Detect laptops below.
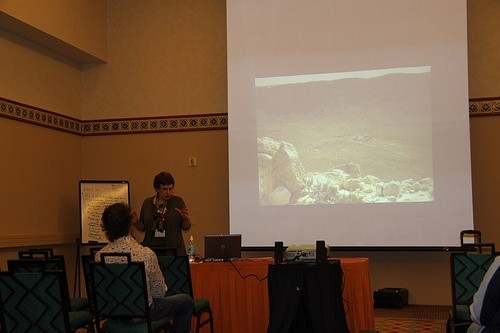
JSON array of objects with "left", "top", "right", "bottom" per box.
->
[{"left": 192, "top": 234, "right": 242, "bottom": 262}]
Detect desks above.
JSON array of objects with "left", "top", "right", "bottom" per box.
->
[{"left": 171, "top": 257, "right": 374, "bottom": 333}]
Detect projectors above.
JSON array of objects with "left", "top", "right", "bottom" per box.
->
[{"left": 285, "top": 243, "right": 329, "bottom": 260}]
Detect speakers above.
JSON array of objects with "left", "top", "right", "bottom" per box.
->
[{"left": 373, "top": 288, "right": 408, "bottom": 309}]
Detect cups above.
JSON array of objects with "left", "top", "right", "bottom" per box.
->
[
  {"left": 315, "top": 240, "right": 326, "bottom": 261},
  {"left": 274, "top": 241, "right": 283, "bottom": 263}
]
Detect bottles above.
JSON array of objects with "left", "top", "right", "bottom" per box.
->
[{"left": 188, "top": 235, "right": 195, "bottom": 262}]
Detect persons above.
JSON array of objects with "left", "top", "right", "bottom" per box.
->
[
  {"left": 131, "top": 171, "right": 191, "bottom": 255},
  {"left": 96, "top": 203, "right": 193, "bottom": 323},
  {"left": 466, "top": 256, "right": 500, "bottom": 333}
]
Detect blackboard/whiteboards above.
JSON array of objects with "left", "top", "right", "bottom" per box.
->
[{"left": 79, "top": 179, "right": 131, "bottom": 246}]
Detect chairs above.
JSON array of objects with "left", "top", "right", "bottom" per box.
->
[
  {"left": 0, "top": 249, "right": 211, "bottom": 333},
  {"left": 451, "top": 251, "right": 500, "bottom": 333}
]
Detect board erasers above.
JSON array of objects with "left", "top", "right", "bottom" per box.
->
[{"left": 89, "top": 240, "right": 98, "bottom": 244}]
